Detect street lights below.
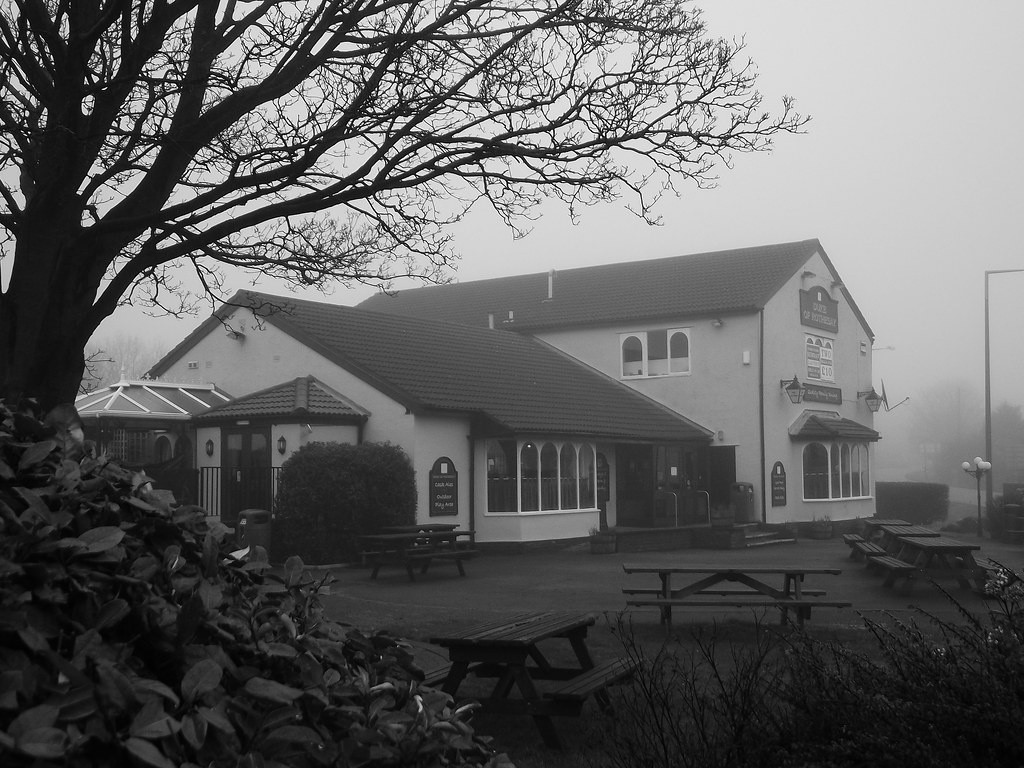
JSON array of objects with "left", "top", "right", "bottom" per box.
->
[{"left": 961, "top": 456, "right": 992, "bottom": 537}]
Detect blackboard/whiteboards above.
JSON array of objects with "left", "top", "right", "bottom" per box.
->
[
  {"left": 771, "top": 461, "right": 787, "bottom": 507},
  {"left": 429, "top": 456, "right": 458, "bottom": 518},
  {"left": 588, "top": 452, "right": 610, "bottom": 501}
]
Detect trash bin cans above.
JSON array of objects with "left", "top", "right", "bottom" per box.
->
[
  {"left": 730, "top": 481, "right": 755, "bottom": 523},
  {"left": 237, "top": 508, "right": 272, "bottom": 563}
]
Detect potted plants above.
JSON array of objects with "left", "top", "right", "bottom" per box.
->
[
  {"left": 810, "top": 515, "right": 833, "bottom": 540},
  {"left": 588, "top": 525, "right": 617, "bottom": 553}
]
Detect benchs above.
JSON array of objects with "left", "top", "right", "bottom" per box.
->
[
  {"left": 546, "top": 654, "right": 647, "bottom": 704},
  {"left": 842, "top": 534, "right": 865, "bottom": 543},
  {"left": 955, "top": 556, "right": 998, "bottom": 572},
  {"left": 622, "top": 586, "right": 826, "bottom": 595},
  {"left": 359, "top": 539, "right": 479, "bottom": 566},
  {"left": 626, "top": 598, "right": 851, "bottom": 609},
  {"left": 854, "top": 542, "right": 887, "bottom": 556},
  {"left": 869, "top": 555, "right": 918, "bottom": 573},
  {"left": 422, "top": 660, "right": 486, "bottom": 687}
]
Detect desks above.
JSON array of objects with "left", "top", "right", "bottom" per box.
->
[
  {"left": 865, "top": 525, "right": 941, "bottom": 579},
  {"left": 364, "top": 530, "right": 477, "bottom": 584},
  {"left": 378, "top": 523, "right": 461, "bottom": 562},
  {"left": 850, "top": 518, "right": 913, "bottom": 562},
  {"left": 622, "top": 562, "right": 842, "bottom": 630},
  {"left": 882, "top": 536, "right": 986, "bottom": 596},
  {"left": 430, "top": 610, "right": 615, "bottom": 751}
]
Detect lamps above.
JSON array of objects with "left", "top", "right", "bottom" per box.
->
[
  {"left": 857, "top": 387, "right": 883, "bottom": 412},
  {"left": 780, "top": 375, "right": 806, "bottom": 404},
  {"left": 226, "top": 330, "right": 245, "bottom": 339},
  {"left": 277, "top": 436, "right": 286, "bottom": 455},
  {"left": 711, "top": 315, "right": 723, "bottom": 327},
  {"left": 206, "top": 438, "right": 214, "bottom": 457}
]
